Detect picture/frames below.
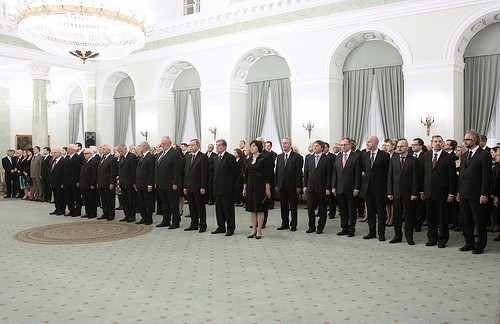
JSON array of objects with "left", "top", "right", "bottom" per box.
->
[{"left": 85, "top": 132, "right": 97, "bottom": 148}]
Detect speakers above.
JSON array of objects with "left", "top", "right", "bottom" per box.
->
[{"left": 85, "top": 132, "right": 96, "bottom": 148}]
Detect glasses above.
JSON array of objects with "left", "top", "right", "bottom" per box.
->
[
  {"left": 464, "top": 138, "right": 472, "bottom": 141},
  {"left": 84, "top": 153, "right": 89, "bottom": 155}
]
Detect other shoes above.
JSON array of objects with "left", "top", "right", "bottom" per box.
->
[
  {"left": 47, "top": 201, "right": 364, "bottom": 239},
  {"left": 362, "top": 225, "right": 500, "bottom": 254},
  {"left": 3, "top": 195, "right": 55, "bottom": 203}
]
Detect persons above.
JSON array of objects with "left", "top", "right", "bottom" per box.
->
[{"left": 2, "top": 129, "right": 500, "bottom": 254}]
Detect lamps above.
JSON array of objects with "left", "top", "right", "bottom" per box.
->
[
  {"left": 302, "top": 123, "right": 315, "bottom": 138},
  {"left": 141, "top": 131, "right": 147, "bottom": 142},
  {"left": 421, "top": 115, "right": 434, "bottom": 135},
  {"left": 0, "top": 0, "right": 157, "bottom": 65},
  {"left": 209, "top": 127, "right": 217, "bottom": 142}
]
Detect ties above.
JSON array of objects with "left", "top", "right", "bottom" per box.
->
[
  {"left": 343, "top": 153, "right": 347, "bottom": 168},
  {"left": 415, "top": 154, "right": 417, "bottom": 158},
  {"left": 86, "top": 160, "right": 88, "bottom": 163},
  {"left": 432, "top": 153, "right": 438, "bottom": 170},
  {"left": 140, "top": 155, "right": 143, "bottom": 162},
  {"left": 191, "top": 155, "right": 195, "bottom": 165},
  {"left": 102, "top": 156, "right": 106, "bottom": 161},
  {"left": 219, "top": 155, "right": 222, "bottom": 164},
  {"left": 54, "top": 159, "right": 57, "bottom": 165},
  {"left": 400, "top": 156, "right": 406, "bottom": 171},
  {"left": 315, "top": 155, "right": 319, "bottom": 168},
  {"left": 159, "top": 152, "right": 165, "bottom": 161},
  {"left": 284, "top": 154, "right": 288, "bottom": 166},
  {"left": 370, "top": 152, "right": 374, "bottom": 166},
  {"left": 468, "top": 151, "right": 472, "bottom": 159}
]
